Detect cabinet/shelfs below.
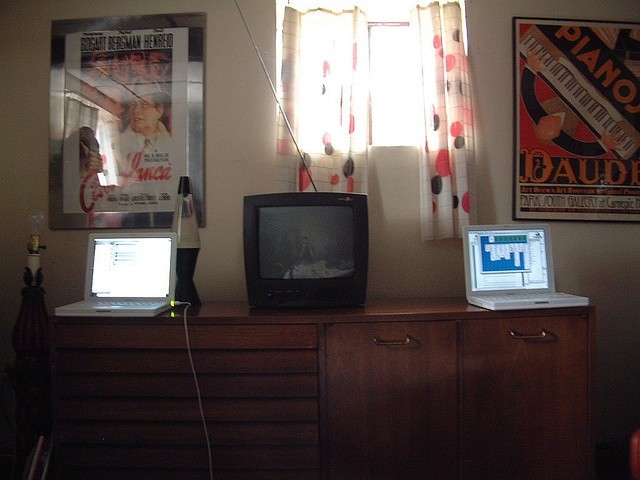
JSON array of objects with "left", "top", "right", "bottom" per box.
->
[
  {"left": 320, "top": 307, "right": 595, "bottom": 478},
  {"left": 52, "top": 318, "right": 319, "bottom": 474}
]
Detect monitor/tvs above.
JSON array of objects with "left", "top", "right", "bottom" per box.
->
[{"left": 244, "top": 193, "right": 370, "bottom": 304}]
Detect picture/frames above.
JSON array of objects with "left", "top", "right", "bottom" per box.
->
[
  {"left": 48, "top": 13, "right": 207, "bottom": 230},
  {"left": 512, "top": 17, "right": 639, "bottom": 224}
]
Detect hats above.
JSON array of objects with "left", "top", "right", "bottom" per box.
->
[{"left": 120, "top": 78, "right": 171, "bottom": 108}]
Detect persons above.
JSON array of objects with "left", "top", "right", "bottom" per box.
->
[
  {"left": 113, "top": 78, "right": 172, "bottom": 157},
  {"left": 93, "top": 55, "right": 113, "bottom": 78},
  {"left": 298, "top": 237, "right": 316, "bottom": 264},
  {"left": 127, "top": 50, "right": 144, "bottom": 73},
  {"left": 149, "top": 54, "right": 170, "bottom": 77}
]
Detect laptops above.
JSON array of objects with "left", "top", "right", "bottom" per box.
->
[
  {"left": 455, "top": 208, "right": 590, "bottom": 310},
  {"left": 54, "top": 235, "right": 182, "bottom": 322}
]
